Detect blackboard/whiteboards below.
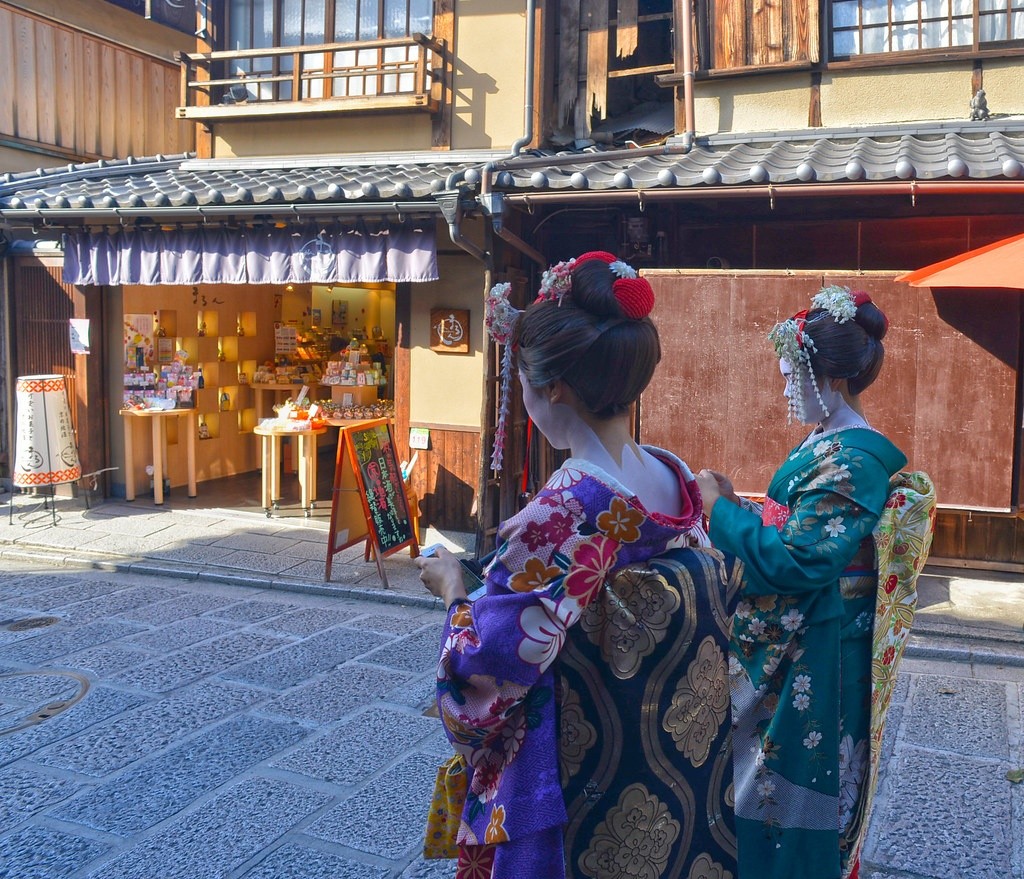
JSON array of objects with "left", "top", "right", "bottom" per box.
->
[{"left": 324, "top": 417, "right": 421, "bottom": 558}]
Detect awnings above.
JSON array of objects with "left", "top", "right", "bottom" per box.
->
[{"left": 894, "top": 234, "right": 1024, "bottom": 289}]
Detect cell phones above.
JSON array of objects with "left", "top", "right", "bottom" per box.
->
[{"left": 422, "top": 543, "right": 487, "bottom": 603}]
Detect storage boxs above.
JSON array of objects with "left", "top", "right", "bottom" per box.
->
[{"left": 331, "top": 385, "right": 377, "bottom": 409}]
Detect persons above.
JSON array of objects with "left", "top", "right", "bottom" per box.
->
[
  {"left": 414, "top": 251, "right": 750, "bottom": 879},
  {"left": 696, "top": 285, "right": 936, "bottom": 879}
]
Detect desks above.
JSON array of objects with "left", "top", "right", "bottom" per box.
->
[
  {"left": 249, "top": 382, "right": 396, "bottom": 518},
  {"left": 119, "top": 407, "right": 199, "bottom": 506}
]
